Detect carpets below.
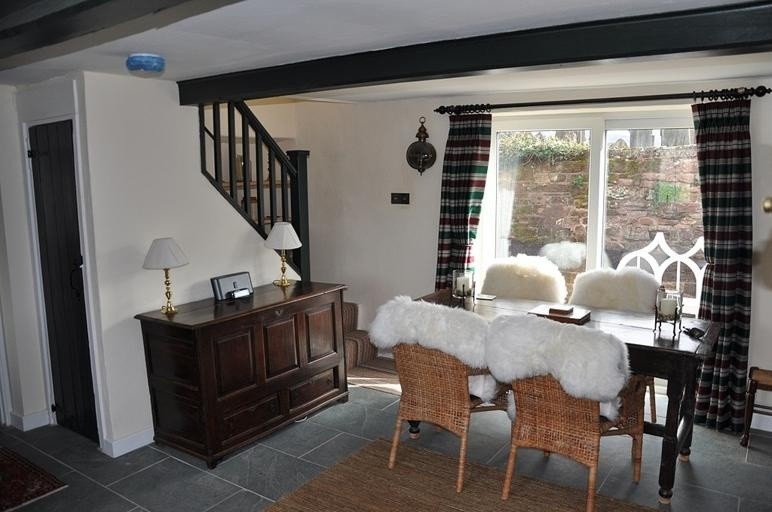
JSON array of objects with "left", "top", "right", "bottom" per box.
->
[
  {"left": 0, "top": 443, "right": 68, "bottom": 510},
  {"left": 263, "top": 437, "right": 633, "bottom": 512}
]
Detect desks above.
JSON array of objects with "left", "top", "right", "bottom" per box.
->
[{"left": 407, "top": 289, "right": 722, "bottom": 505}]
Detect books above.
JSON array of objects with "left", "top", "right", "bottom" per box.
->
[{"left": 527, "top": 304, "right": 591, "bottom": 325}]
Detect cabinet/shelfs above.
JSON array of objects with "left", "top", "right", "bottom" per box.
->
[{"left": 135, "top": 280, "right": 351, "bottom": 469}]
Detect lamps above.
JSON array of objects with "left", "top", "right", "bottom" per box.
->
[
  {"left": 143, "top": 238, "right": 189, "bottom": 315},
  {"left": 264, "top": 220, "right": 303, "bottom": 285},
  {"left": 406, "top": 118, "right": 435, "bottom": 174}
]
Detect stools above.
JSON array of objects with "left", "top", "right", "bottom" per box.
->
[{"left": 740, "top": 367, "right": 772, "bottom": 447}]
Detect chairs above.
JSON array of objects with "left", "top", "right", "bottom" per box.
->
[
  {"left": 487, "top": 312, "right": 647, "bottom": 511},
  {"left": 482, "top": 259, "right": 568, "bottom": 303},
  {"left": 369, "top": 296, "right": 516, "bottom": 494},
  {"left": 571, "top": 267, "right": 663, "bottom": 423}
]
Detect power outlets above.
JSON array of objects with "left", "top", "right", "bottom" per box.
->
[{"left": 391, "top": 192, "right": 409, "bottom": 204}]
